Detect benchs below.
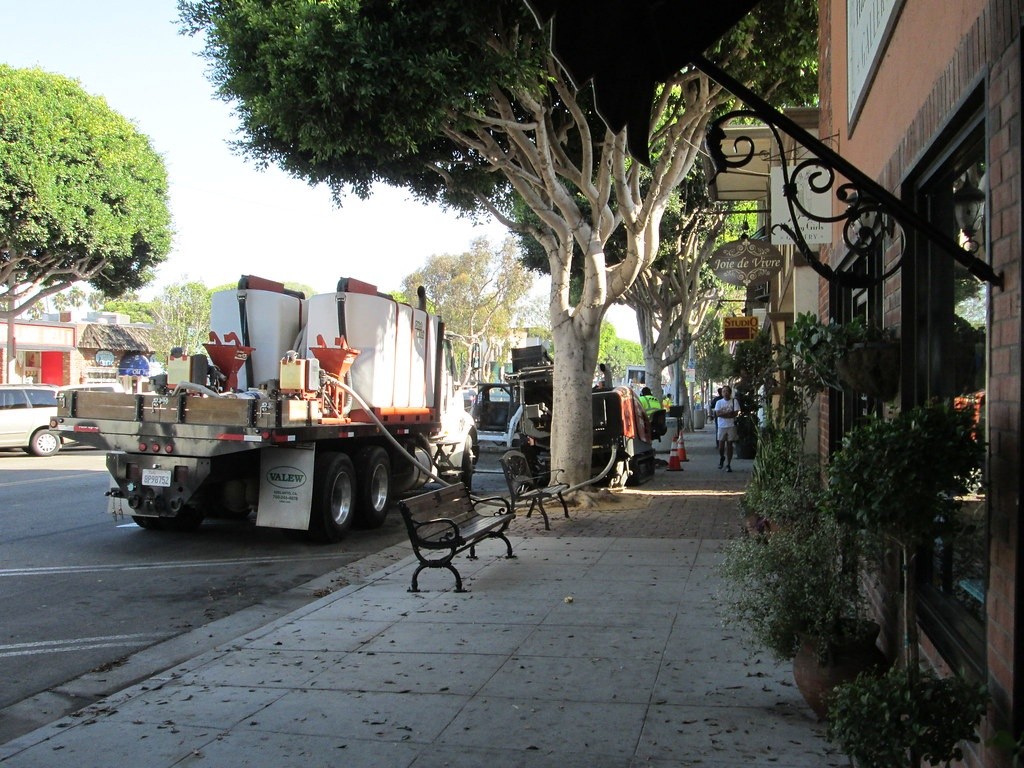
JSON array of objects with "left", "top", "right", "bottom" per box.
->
[
  {"left": 499, "top": 449, "right": 570, "bottom": 531},
  {"left": 397, "top": 482, "right": 516, "bottom": 592}
]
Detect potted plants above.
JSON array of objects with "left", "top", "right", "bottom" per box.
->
[
  {"left": 744, "top": 484, "right": 819, "bottom": 545},
  {"left": 772, "top": 310, "right": 901, "bottom": 399},
  {"left": 712, "top": 538, "right": 887, "bottom": 720}
]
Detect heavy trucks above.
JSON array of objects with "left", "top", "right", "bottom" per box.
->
[{"left": 47, "top": 275, "right": 481, "bottom": 545}]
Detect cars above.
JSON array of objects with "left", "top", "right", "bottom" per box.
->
[
  {"left": 0, "top": 384, "right": 83, "bottom": 459},
  {"left": 458, "top": 340, "right": 679, "bottom": 496},
  {"left": 56, "top": 380, "right": 129, "bottom": 407}
]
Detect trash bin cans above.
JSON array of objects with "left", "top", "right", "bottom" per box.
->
[
  {"left": 694, "top": 409, "right": 704, "bottom": 429},
  {"left": 651, "top": 416, "right": 678, "bottom": 454}
]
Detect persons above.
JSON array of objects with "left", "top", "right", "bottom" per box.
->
[
  {"left": 694, "top": 393, "right": 701, "bottom": 409},
  {"left": 661, "top": 389, "right": 672, "bottom": 416},
  {"left": 714, "top": 386, "right": 742, "bottom": 472},
  {"left": 639, "top": 387, "right": 661, "bottom": 422},
  {"left": 711, "top": 388, "right": 723, "bottom": 449}
]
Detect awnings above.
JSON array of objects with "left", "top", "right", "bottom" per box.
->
[{"left": 520, "top": 0, "right": 1006, "bottom": 293}]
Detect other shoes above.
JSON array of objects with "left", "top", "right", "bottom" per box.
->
[
  {"left": 725, "top": 466, "right": 731, "bottom": 472},
  {"left": 718, "top": 455, "right": 725, "bottom": 469}
]
